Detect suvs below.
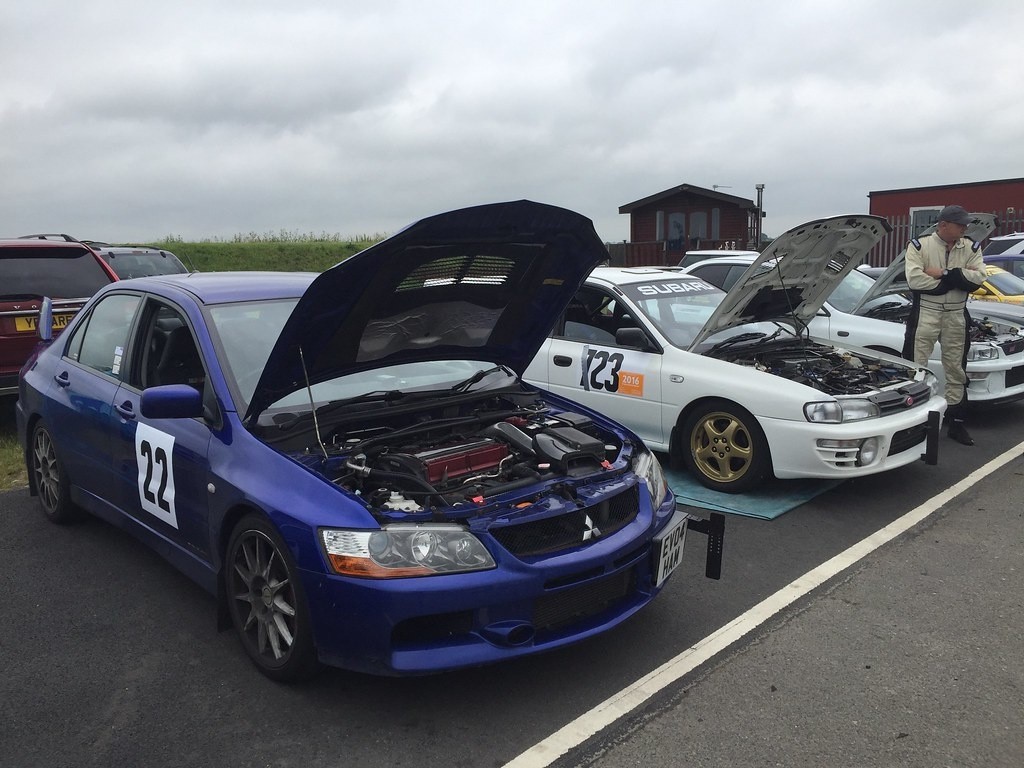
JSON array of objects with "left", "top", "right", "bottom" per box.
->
[{"left": 0, "top": 232, "right": 129, "bottom": 398}]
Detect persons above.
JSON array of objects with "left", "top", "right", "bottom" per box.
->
[{"left": 902, "top": 205, "right": 989, "bottom": 446}]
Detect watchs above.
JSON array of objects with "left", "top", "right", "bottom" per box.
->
[{"left": 942, "top": 269, "right": 949, "bottom": 276}]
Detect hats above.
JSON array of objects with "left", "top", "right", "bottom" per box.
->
[{"left": 936, "top": 205, "right": 974, "bottom": 225}]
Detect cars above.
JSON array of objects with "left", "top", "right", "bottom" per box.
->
[
  {"left": 13, "top": 198, "right": 691, "bottom": 687},
  {"left": 861, "top": 232, "right": 1024, "bottom": 308},
  {"left": 83, "top": 239, "right": 189, "bottom": 282},
  {"left": 423, "top": 212, "right": 949, "bottom": 495},
  {"left": 663, "top": 211, "right": 1024, "bottom": 418},
  {"left": 674, "top": 248, "right": 757, "bottom": 276}
]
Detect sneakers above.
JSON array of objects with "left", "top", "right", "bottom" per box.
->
[{"left": 947, "top": 424, "right": 974, "bottom": 445}]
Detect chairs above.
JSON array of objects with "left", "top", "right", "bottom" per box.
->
[{"left": 155, "top": 326, "right": 205, "bottom": 392}]
